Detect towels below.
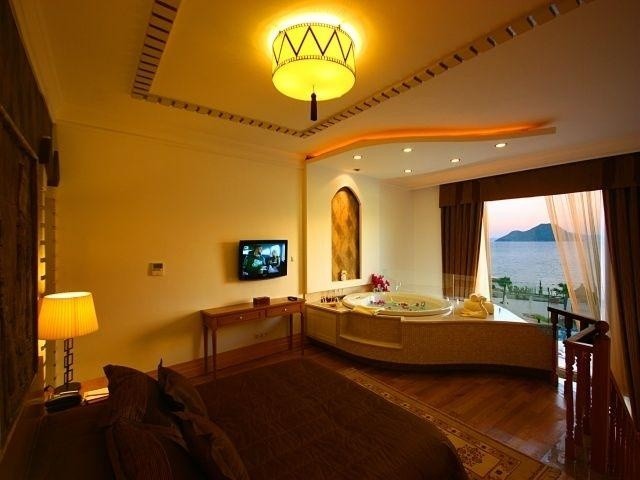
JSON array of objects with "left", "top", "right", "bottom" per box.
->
[{"left": 454, "top": 293, "right": 494, "bottom": 319}]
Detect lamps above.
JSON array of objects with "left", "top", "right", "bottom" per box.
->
[
  {"left": 268, "top": 22, "right": 362, "bottom": 122},
  {"left": 38, "top": 290, "right": 99, "bottom": 395}
]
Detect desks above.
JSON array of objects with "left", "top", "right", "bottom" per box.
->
[{"left": 199, "top": 295, "right": 307, "bottom": 382}]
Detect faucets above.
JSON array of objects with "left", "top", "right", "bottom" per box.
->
[
  {"left": 444, "top": 295, "right": 449, "bottom": 302},
  {"left": 454, "top": 297, "right": 460, "bottom": 305}
]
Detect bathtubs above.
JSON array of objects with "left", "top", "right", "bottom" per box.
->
[{"left": 342, "top": 291, "right": 451, "bottom": 316}]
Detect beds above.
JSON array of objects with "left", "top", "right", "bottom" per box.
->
[{"left": 1, "top": 355, "right": 468, "bottom": 480}]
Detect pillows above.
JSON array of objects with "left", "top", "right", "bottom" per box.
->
[{"left": 96, "top": 357, "right": 249, "bottom": 480}]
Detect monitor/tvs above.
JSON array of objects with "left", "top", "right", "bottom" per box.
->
[{"left": 238, "top": 239, "right": 288, "bottom": 280}]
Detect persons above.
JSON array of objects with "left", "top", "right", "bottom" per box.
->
[
  {"left": 243, "top": 245, "right": 268, "bottom": 275},
  {"left": 269, "top": 250, "right": 282, "bottom": 269}
]
